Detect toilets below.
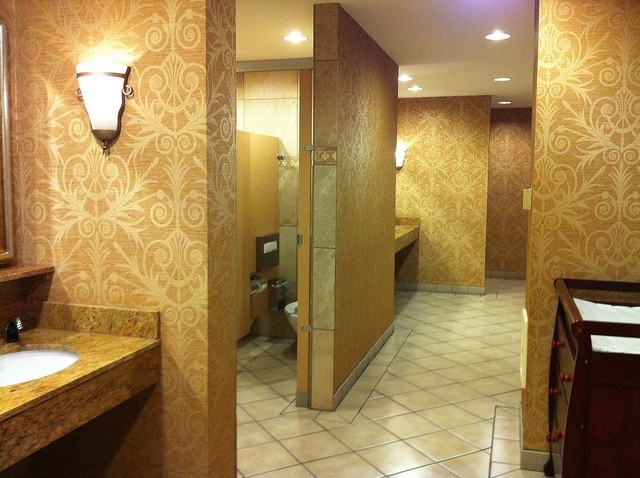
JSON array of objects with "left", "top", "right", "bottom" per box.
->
[{"left": 284, "top": 300, "right": 297, "bottom": 337}]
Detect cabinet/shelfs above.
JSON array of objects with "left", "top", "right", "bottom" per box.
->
[{"left": 543, "top": 278, "right": 640, "bottom": 477}]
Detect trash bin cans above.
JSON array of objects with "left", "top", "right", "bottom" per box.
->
[{"left": 268, "top": 278, "right": 289, "bottom": 312}]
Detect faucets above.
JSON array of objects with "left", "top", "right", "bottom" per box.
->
[{"left": 5, "top": 315, "right": 24, "bottom": 343}]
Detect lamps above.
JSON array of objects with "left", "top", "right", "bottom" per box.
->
[
  {"left": 395, "top": 150, "right": 406, "bottom": 170},
  {"left": 75, "top": 59, "right": 131, "bottom": 156}
]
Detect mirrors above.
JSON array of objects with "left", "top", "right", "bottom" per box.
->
[{"left": 0, "top": 25, "right": 15, "bottom": 266}]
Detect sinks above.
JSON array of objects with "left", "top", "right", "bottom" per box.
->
[{"left": 0, "top": 343, "right": 80, "bottom": 389}]
